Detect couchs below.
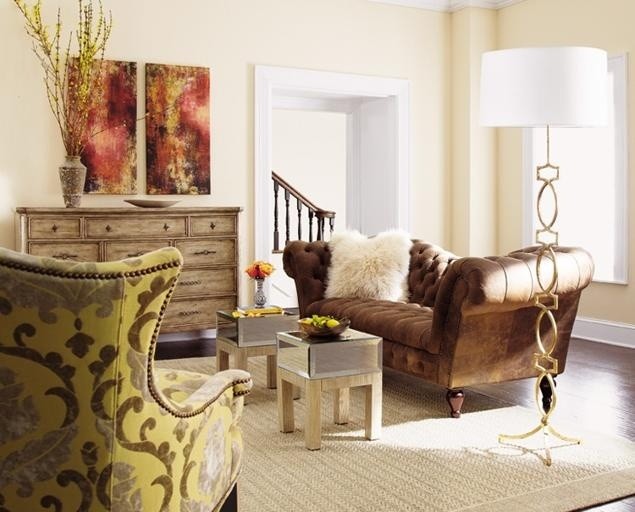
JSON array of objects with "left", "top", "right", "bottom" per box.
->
[{"left": 283, "top": 235, "right": 594, "bottom": 418}]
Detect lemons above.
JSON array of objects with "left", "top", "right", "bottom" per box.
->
[
  {"left": 326, "top": 320, "right": 340, "bottom": 328},
  {"left": 301, "top": 319, "right": 312, "bottom": 328}
]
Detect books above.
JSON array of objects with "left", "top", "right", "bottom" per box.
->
[{"left": 238, "top": 304, "right": 284, "bottom": 315}]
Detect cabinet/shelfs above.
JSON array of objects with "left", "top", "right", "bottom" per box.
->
[{"left": 16, "top": 206, "right": 244, "bottom": 335}]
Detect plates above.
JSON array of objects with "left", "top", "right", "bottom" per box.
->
[{"left": 123, "top": 200, "right": 180, "bottom": 208}]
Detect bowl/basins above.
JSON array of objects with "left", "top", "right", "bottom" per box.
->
[{"left": 297, "top": 317, "right": 351, "bottom": 338}]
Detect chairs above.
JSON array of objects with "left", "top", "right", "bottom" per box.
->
[{"left": 0, "top": 246, "right": 253, "bottom": 512}]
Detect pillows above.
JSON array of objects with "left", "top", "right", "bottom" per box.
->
[{"left": 324, "top": 228, "right": 411, "bottom": 305}]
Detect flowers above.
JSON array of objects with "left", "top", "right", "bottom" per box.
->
[
  {"left": 244, "top": 261, "right": 274, "bottom": 280},
  {"left": 14, "top": 0, "right": 184, "bottom": 156}
]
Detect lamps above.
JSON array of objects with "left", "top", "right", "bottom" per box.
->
[{"left": 480, "top": 46, "right": 608, "bottom": 464}]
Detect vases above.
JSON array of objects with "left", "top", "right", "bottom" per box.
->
[
  {"left": 255, "top": 280, "right": 266, "bottom": 308},
  {"left": 58, "top": 156, "right": 88, "bottom": 208}
]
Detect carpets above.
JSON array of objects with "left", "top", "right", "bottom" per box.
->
[{"left": 152, "top": 341, "right": 635, "bottom": 512}]
